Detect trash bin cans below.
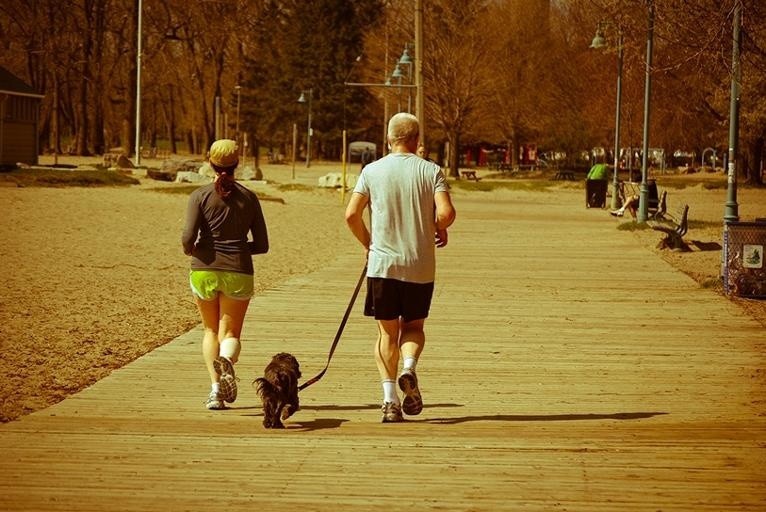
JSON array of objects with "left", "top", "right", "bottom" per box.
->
[{"left": 725, "top": 221, "right": 766, "bottom": 300}]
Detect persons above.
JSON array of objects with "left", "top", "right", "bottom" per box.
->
[
  {"left": 417, "top": 142, "right": 452, "bottom": 190},
  {"left": 361, "top": 147, "right": 372, "bottom": 168},
  {"left": 345, "top": 112, "right": 456, "bottom": 422},
  {"left": 585, "top": 162, "right": 610, "bottom": 208},
  {"left": 609, "top": 176, "right": 659, "bottom": 222},
  {"left": 181, "top": 139, "right": 268, "bottom": 408}
]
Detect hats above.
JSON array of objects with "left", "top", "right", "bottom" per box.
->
[{"left": 209, "top": 139, "right": 239, "bottom": 168}]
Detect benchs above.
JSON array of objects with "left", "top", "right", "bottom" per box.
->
[
  {"left": 555, "top": 171, "right": 575, "bottom": 181},
  {"left": 461, "top": 171, "right": 478, "bottom": 179},
  {"left": 606, "top": 182, "right": 693, "bottom": 252}
]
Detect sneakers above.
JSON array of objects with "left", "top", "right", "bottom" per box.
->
[
  {"left": 213, "top": 356, "right": 237, "bottom": 403},
  {"left": 206, "top": 393, "right": 225, "bottom": 409},
  {"left": 381, "top": 403, "right": 403, "bottom": 422},
  {"left": 398, "top": 371, "right": 422, "bottom": 415}
]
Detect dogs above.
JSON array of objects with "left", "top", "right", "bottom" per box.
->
[{"left": 251, "top": 351, "right": 302, "bottom": 430}]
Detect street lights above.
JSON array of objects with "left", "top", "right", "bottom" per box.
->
[
  {"left": 590, "top": 19, "right": 626, "bottom": 216},
  {"left": 392, "top": 63, "right": 410, "bottom": 113},
  {"left": 399, "top": 44, "right": 413, "bottom": 116},
  {"left": 384, "top": 77, "right": 396, "bottom": 86},
  {"left": 297, "top": 88, "right": 314, "bottom": 170}
]
